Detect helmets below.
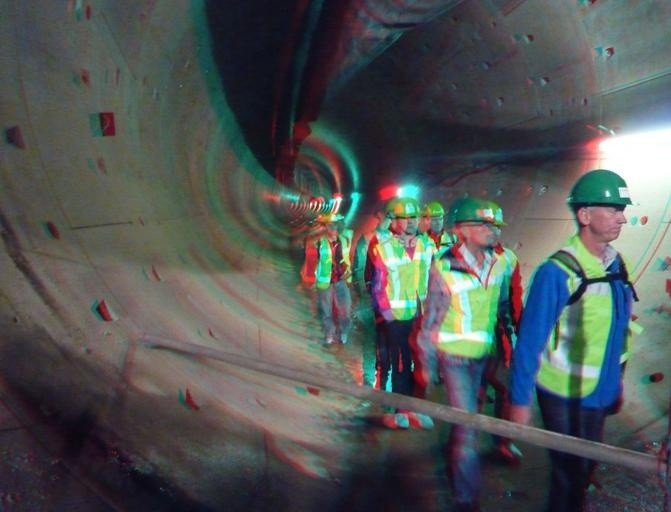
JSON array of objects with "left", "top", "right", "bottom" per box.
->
[
  {"left": 566, "top": 170, "right": 632, "bottom": 205},
  {"left": 319, "top": 213, "right": 344, "bottom": 223},
  {"left": 373, "top": 197, "right": 506, "bottom": 225}
]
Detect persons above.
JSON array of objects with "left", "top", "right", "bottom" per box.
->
[{"left": 299, "top": 169, "right": 639, "bottom": 511}]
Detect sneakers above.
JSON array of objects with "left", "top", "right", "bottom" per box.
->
[
  {"left": 498, "top": 440, "right": 523, "bottom": 461},
  {"left": 326, "top": 334, "right": 348, "bottom": 344},
  {"left": 384, "top": 412, "right": 434, "bottom": 428}
]
